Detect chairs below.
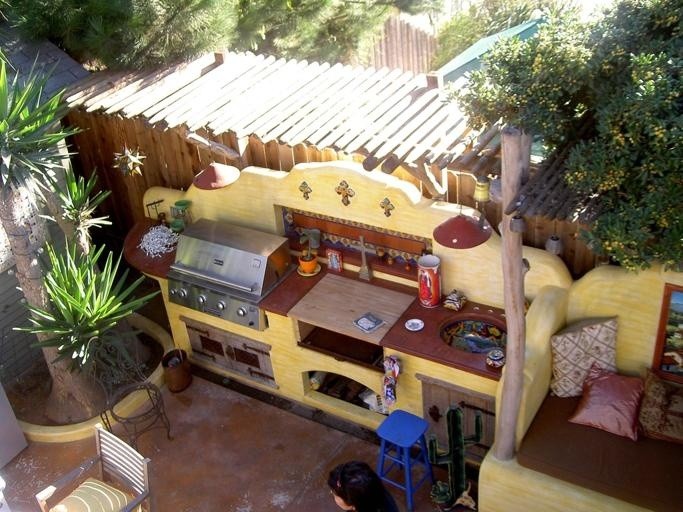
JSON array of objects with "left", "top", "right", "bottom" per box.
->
[{"left": 34, "top": 422, "right": 156, "bottom": 512}]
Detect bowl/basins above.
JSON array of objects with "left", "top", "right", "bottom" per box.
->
[{"left": 169, "top": 220, "right": 183, "bottom": 232}]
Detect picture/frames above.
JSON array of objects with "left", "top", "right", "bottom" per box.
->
[{"left": 650, "top": 282, "right": 683, "bottom": 387}]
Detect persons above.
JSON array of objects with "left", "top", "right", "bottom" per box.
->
[{"left": 328, "top": 460, "right": 399, "bottom": 511}]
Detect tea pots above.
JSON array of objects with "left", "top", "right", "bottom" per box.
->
[
  {"left": 486, "top": 350, "right": 505, "bottom": 370},
  {"left": 417, "top": 255, "right": 441, "bottom": 310}
]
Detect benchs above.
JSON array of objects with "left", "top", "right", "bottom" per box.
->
[{"left": 477, "top": 254, "right": 682, "bottom": 511}]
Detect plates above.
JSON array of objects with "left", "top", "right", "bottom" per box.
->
[{"left": 404, "top": 317, "right": 424, "bottom": 333}]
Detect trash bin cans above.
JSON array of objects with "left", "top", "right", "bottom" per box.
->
[{"left": 161, "top": 349, "right": 192, "bottom": 394}]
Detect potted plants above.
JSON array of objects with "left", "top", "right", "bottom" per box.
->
[{"left": 298, "top": 226, "right": 322, "bottom": 276}]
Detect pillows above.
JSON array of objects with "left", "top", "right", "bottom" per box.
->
[{"left": 552, "top": 313, "right": 683, "bottom": 444}]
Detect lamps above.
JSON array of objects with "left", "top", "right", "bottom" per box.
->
[
  {"left": 432, "top": 175, "right": 491, "bottom": 248},
  {"left": 193, "top": 127, "right": 241, "bottom": 190}
]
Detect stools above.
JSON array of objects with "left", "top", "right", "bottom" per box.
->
[{"left": 375, "top": 410, "right": 437, "bottom": 512}]
